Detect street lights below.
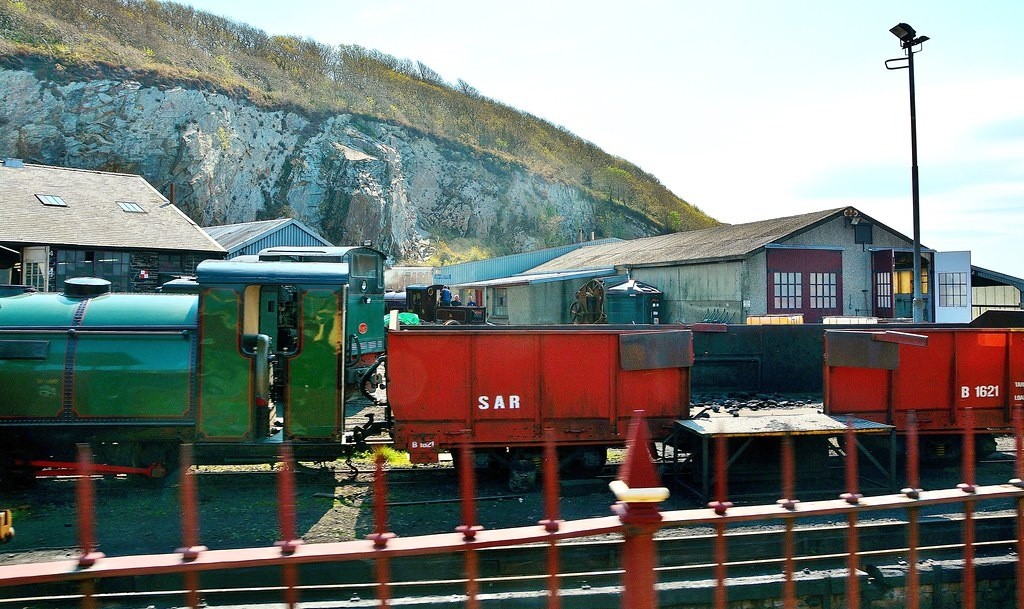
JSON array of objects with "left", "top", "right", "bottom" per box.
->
[{"left": 883, "top": 22, "right": 930, "bottom": 326}]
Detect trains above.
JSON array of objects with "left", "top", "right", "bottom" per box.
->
[
  {"left": 384, "top": 284, "right": 488, "bottom": 325},
  {"left": 1, "top": 257, "right": 1023, "bottom": 490}
]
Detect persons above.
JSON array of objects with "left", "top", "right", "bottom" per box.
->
[
  {"left": 467, "top": 295, "right": 476, "bottom": 307},
  {"left": 451, "top": 294, "right": 462, "bottom": 306},
  {"left": 438, "top": 285, "right": 452, "bottom": 307}
]
